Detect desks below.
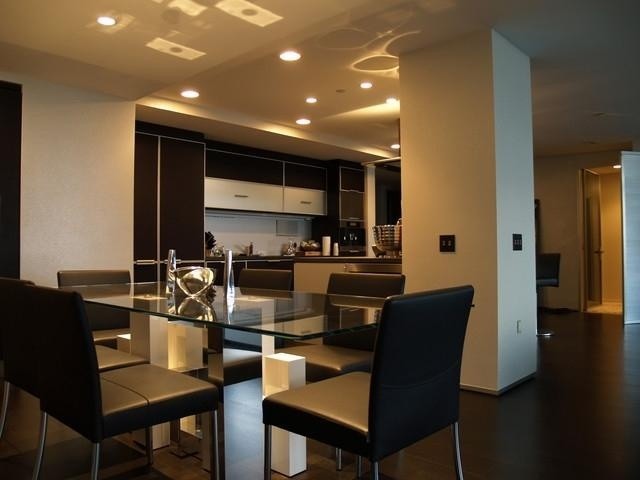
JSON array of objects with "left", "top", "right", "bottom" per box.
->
[{"left": 79, "top": 286, "right": 408, "bottom": 479}]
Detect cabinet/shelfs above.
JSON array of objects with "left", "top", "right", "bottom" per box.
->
[
  {"left": 206, "top": 146, "right": 284, "bottom": 214},
  {"left": 310, "top": 159, "right": 365, "bottom": 253},
  {"left": 134, "top": 132, "right": 206, "bottom": 283},
  {"left": 283, "top": 157, "right": 328, "bottom": 218}
]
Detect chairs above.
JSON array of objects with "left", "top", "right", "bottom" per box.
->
[
  {"left": 537, "top": 249, "right": 562, "bottom": 338},
  {"left": 54, "top": 265, "right": 138, "bottom": 345},
  {"left": 274, "top": 270, "right": 408, "bottom": 471},
  {"left": 29, "top": 287, "right": 223, "bottom": 479},
  {"left": 261, "top": 285, "right": 480, "bottom": 479},
  {"left": 1, "top": 277, "right": 147, "bottom": 478},
  {"left": 207, "top": 267, "right": 293, "bottom": 387}
]
